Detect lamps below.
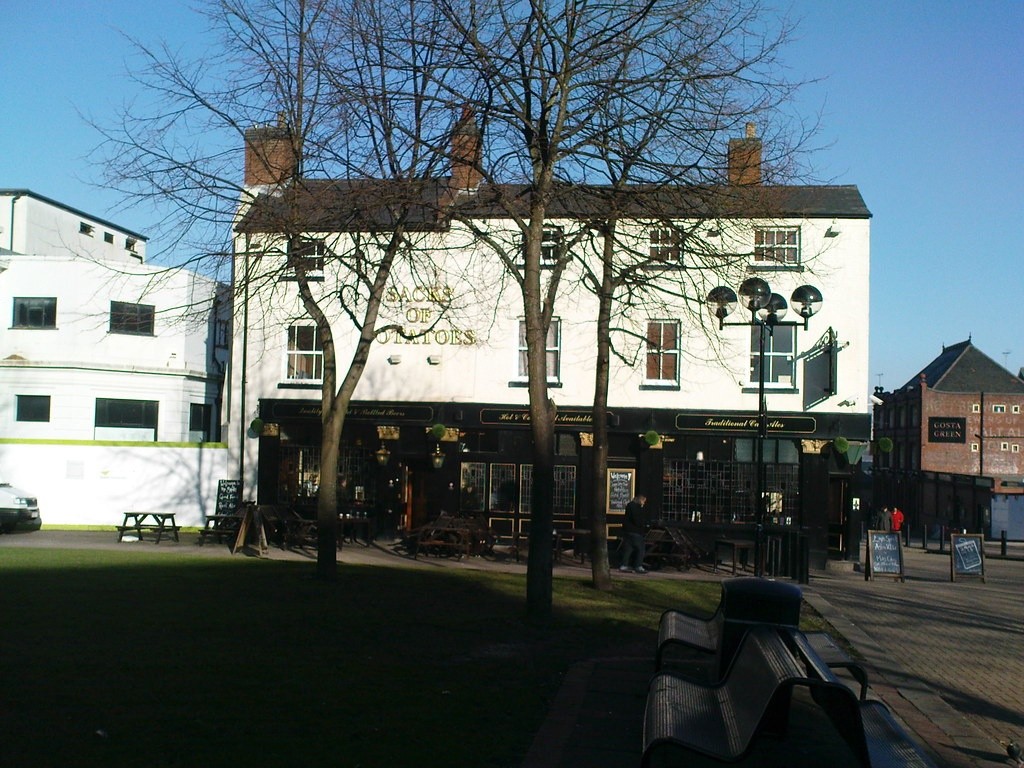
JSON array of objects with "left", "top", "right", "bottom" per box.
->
[
  {"left": 391, "top": 355, "right": 401, "bottom": 363},
  {"left": 429, "top": 355, "right": 442, "bottom": 364},
  {"left": 432, "top": 442, "right": 446, "bottom": 469},
  {"left": 376, "top": 440, "right": 391, "bottom": 466}
]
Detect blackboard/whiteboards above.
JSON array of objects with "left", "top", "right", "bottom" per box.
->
[
  {"left": 949, "top": 533, "right": 985, "bottom": 578},
  {"left": 607, "top": 466, "right": 636, "bottom": 515},
  {"left": 866, "top": 530, "right": 906, "bottom": 577},
  {"left": 215, "top": 478, "right": 242, "bottom": 518}
]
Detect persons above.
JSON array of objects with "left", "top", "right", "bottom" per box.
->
[
  {"left": 618, "top": 494, "right": 650, "bottom": 574},
  {"left": 875, "top": 505, "right": 893, "bottom": 531},
  {"left": 889, "top": 506, "right": 904, "bottom": 531}
]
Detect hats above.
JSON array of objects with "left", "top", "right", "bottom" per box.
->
[{"left": 635, "top": 492, "right": 648, "bottom": 498}]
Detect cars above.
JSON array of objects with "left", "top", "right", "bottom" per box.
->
[{"left": 1, "top": 481, "right": 41, "bottom": 534}]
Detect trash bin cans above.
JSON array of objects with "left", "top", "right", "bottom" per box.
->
[
  {"left": 764, "top": 528, "right": 812, "bottom": 578},
  {"left": 715, "top": 579, "right": 801, "bottom": 698}
]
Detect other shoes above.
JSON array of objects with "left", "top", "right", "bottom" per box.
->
[{"left": 617, "top": 564, "right": 648, "bottom": 573}]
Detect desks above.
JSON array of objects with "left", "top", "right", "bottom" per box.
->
[
  {"left": 512, "top": 532, "right": 529, "bottom": 562},
  {"left": 714, "top": 540, "right": 756, "bottom": 575},
  {"left": 199, "top": 515, "right": 243, "bottom": 546},
  {"left": 556, "top": 529, "right": 591, "bottom": 564},
  {"left": 118, "top": 513, "right": 180, "bottom": 544},
  {"left": 337, "top": 518, "right": 373, "bottom": 550}
]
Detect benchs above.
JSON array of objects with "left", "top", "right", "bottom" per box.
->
[
  {"left": 115, "top": 526, "right": 182, "bottom": 543},
  {"left": 641, "top": 579, "right": 937, "bottom": 767},
  {"left": 198, "top": 526, "right": 237, "bottom": 546}
]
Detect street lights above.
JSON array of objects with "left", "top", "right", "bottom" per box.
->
[{"left": 708, "top": 276, "right": 825, "bottom": 577}]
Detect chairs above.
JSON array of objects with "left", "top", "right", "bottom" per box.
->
[
  {"left": 260, "top": 503, "right": 530, "bottom": 562},
  {"left": 553, "top": 519, "right": 756, "bottom": 582}
]
